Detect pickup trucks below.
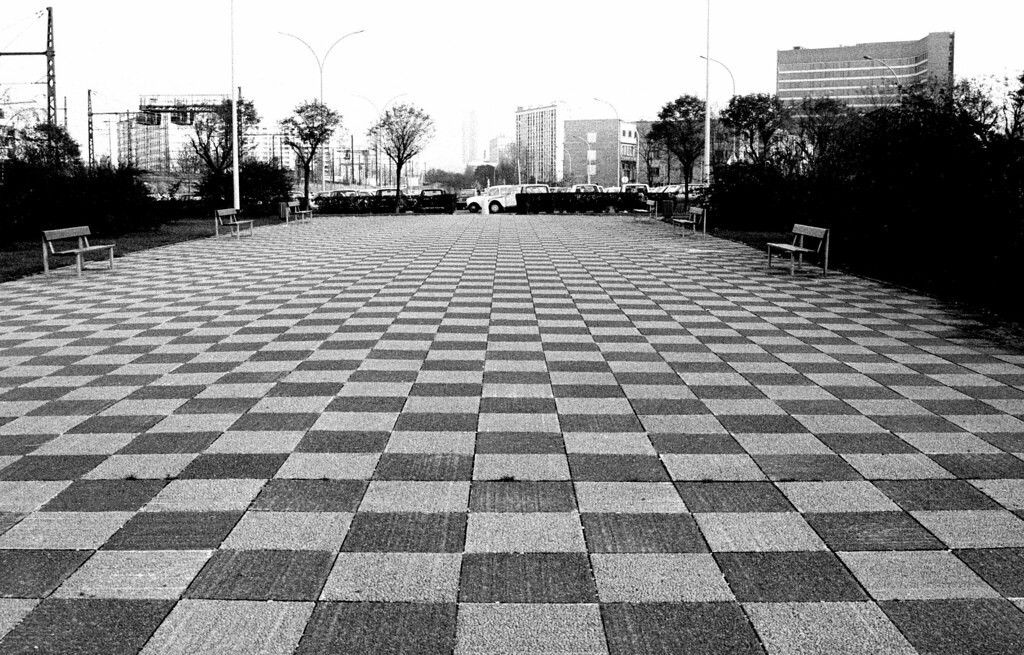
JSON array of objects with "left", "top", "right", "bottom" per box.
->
[
  {"left": 456, "top": 188, "right": 480, "bottom": 210},
  {"left": 412, "top": 189, "right": 457, "bottom": 212}
]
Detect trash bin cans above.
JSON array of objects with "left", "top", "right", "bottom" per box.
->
[
  {"left": 660, "top": 199, "right": 672, "bottom": 223},
  {"left": 278, "top": 202, "right": 287, "bottom": 221}
]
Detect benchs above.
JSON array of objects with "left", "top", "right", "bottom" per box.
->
[
  {"left": 42, "top": 226, "right": 115, "bottom": 279},
  {"left": 285, "top": 201, "right": 313, "bottom": 225},
  {"left": 766, "top": 223, "right": 829, "bottom": 278},
  {"left": 672, "top": 207, "right": 706, "bottom": 240},
  {"left": 633, "top": 199, "right": 657, "bottom": 225},
  {"left": 215, "top": 208, "right": 255, "bottom": 240}
]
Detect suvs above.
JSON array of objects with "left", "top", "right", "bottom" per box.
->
[{"left": 488, "top": 184, "right": 551, "bottom": 213}]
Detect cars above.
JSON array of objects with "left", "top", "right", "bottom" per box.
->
[
  {"left": 405, "top": 195, "right": 419, "bottom": 202},
  {"left": 373, "top": 189, "right": 408, "bottom": 213},
  {"left": 316, "top": 191, "right": 330, "bottom": 198},
  {"left": 466, "top": 185, "right": 516, "bottom": 213},
  {"left": 549, "top": 184, "right": 712, "bottom": 210},
  {"left": 286, "top": 191, "right": 304, "bottom": 206},
  {"left": 330, "top": 189, "right": 374, "bottom": 212}
]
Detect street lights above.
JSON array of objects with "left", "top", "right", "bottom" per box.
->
[
  {"left": 277, "top": 29, "right": 366, "bottom": 192},
  {"left": 618, "top": 118, "right": 639, "bottom": 184},
  {"left": 355, "top": 93, "right": 408, "bottom": 188},
  {"left": 863, "top": 54, "right": 902, "bottom": 112},
  {"left": 572, "top": 135, "right": 591, "bottom": 184},
  {"left": 700, "top": 55, "right": 738, "bottom": 165},
  {"left": 593, "top": 97, "right": 620, "bottom": 187}
]
